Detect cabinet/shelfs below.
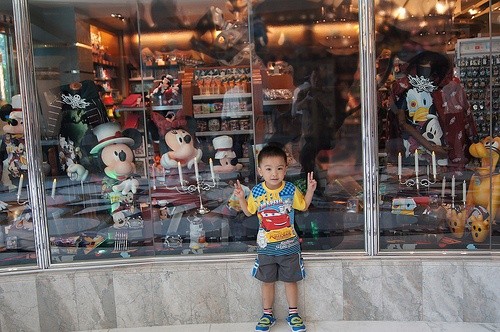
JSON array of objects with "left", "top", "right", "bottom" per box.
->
[
  {"left": 182, "top": 24, "right": 297, "bottom": 184},
  {"left": 128, "top": 58, "right": 183, "bottom": 122},
  {"left": 86, "top": 23, "right": 121, "bottom": 120}
]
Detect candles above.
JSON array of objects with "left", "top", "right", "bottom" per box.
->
[
  {"left": 462, "top": 179, "right": 467, "bottom": 200},
  {"left": 414, "top": 148, "right": 419, "bottom": 172},
  {"left": 432, "top": 151, "right": 437, "bottom": 174},
  {"left": 451, "top": 174, "right": 456, "bottom": 195},
  {"left": 441, "top": 176, "right": 446, "bottom": 194},
  {"left": 397, "top": 152, "right": 402, "bottom": 175}
]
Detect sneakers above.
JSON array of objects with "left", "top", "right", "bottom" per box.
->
[
  {"left": 255, "top": 315, "right": 276, "bottom": 332},
  {"left": 286, "top": 314, "right": 306, "bottom": 332}
]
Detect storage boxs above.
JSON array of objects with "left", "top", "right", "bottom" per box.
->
[
  {"left": 120, "top": 94, "right": 142, "bottom": 108},
  {"left": 192, "top": 102, "right": 250, "bottom": 131}
]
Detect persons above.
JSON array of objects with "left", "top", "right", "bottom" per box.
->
[
  {"left": 296, "top": 67, "right": 342, "bottom": 196},
  {"left": 233, "top": 146, "right": 317, "bottom": 332},
  {"left": 292, "top": 74, "right": 310, "bottom": 138}
]
